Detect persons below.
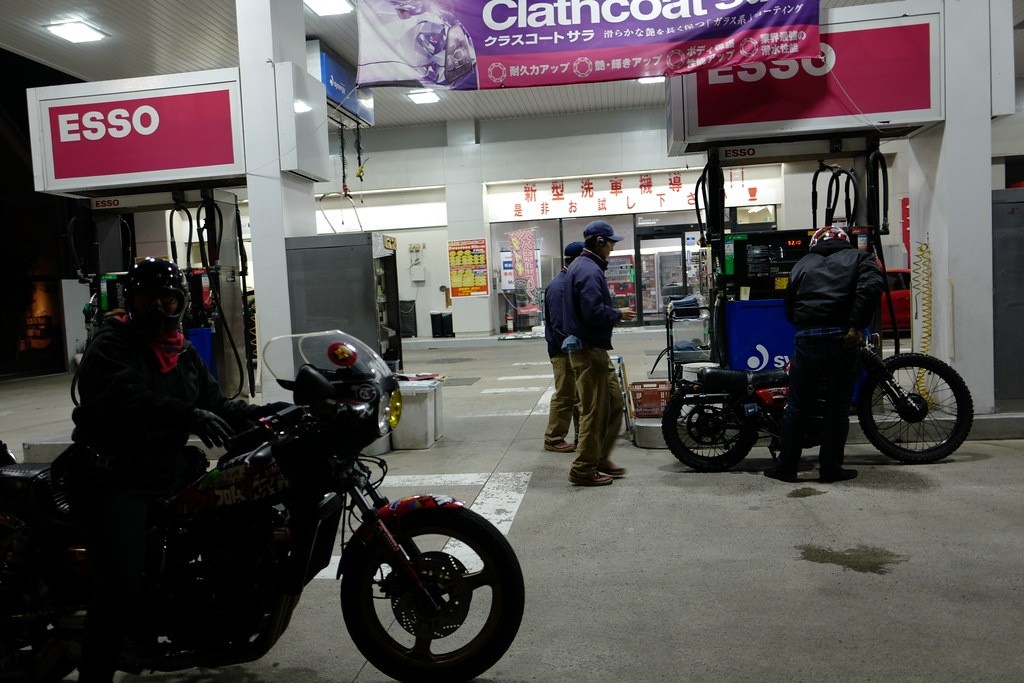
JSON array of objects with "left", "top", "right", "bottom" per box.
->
[
  {"left": 564, "top": 220, "right": 637, "bottom": 487},
  {"left": 544, "top": 242, "right": 586, "bottom": 453},
  {"left": 764, "top": 225, "right": 884, "bottom": 483},
  {"left": 63, "top": 258, "right": 294, "bottom": 683}
]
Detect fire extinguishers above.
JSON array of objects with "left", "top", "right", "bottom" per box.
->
[{"left": 505, "top": 309, "right": 513, "bottom": 333}]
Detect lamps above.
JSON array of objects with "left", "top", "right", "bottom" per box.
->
[
  {"left": 406, "top": 88, "right": 443, "bottom": 105},
  {"left": 303, "top": 0, "right": 356, "bottom": 17},
  {"left": 42, "top": 20, "right": 112, "bottom": 44}
]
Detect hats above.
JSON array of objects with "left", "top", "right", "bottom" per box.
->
[
  {"left": 583, "top": 220, "right": 624, "bottom": 242},
  {"left": 564, "top": 242, "right": 586, "bottom": 258}
]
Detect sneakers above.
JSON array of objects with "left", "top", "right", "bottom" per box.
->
[
  {"left": 544, "top": 440, "right": 574, "bottom": 452},
  {"left": 596, "top": 461, "right": 627, "bottom": 477},
  {"left": 567, "top": 469, "right": 613, "bottom": 485}
]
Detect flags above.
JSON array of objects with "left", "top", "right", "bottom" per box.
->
[{"left": 509, "top": 227, "right": 543, "bottom": 315}]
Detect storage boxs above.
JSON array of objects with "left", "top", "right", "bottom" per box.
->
[{"left": 629, "top": 380, "right": 672, "bottom": 418}]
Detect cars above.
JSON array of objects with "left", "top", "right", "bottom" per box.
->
[{"left": 876, "top": 269, "right": 911, "bottom": 333}]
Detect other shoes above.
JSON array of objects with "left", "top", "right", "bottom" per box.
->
[
  {"left": 764, "top": 466, "right": 795, "bottom": 482},
  {"left": 820, "top": 467, "right": 857, "bottom": 482}
]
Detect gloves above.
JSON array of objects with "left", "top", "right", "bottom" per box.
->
[
  {"left": 836, "top": 327, "right": 863, "bottom": 348},
  {"left": 177, "top": 407, "right": 235, "bottom": 448}
]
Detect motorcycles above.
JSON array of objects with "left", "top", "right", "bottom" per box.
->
[
  {"left": 661, "top": 331, "right": 974, "bottom": 471},
  {"left": 0, "top": 330, "right": 526, "bottom": 683}
]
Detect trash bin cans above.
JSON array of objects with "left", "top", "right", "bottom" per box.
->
[
  {"left": 388, "top": 370, "right": 447, "bottom": 450},
  {"left": 430, "top": 311, "right": 455, "bottom": 338}
]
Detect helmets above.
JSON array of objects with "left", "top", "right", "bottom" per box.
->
[
  {"left": 122, "top": 259, "right": 189, "bottom": 328},
  {"left": 809, "top": 226, "right": 850, "bottom": 253}
]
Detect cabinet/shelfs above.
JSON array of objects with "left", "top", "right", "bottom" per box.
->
[{"left": 666, "top": 306, "right": 715, "bottom": 393}]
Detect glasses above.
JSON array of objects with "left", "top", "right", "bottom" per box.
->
[{"left": 135, "top": 292, "right": 179, "bottom": 303}]
